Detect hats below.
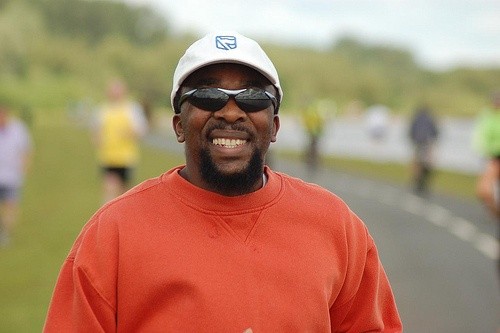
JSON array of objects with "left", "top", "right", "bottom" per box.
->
[{"left": 170, "top": 30, "right": 283, "bottom": 112}]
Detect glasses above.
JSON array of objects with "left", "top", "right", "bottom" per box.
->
[{"left": 178, "top": 87, "right": 278, "bottom": 113}]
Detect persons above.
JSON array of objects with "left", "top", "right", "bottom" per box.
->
[
  {"left": 300, "top": 97, "right": 325, "bottom": 171},
  {"left": 40, "top": 32, "right": 403, "bottom": 333},
  {"left": 473, "top": 90, "right": 500, "bottom": 221},
  {"left": 409, "top": 97, "right": 439, "bottom": 196},
  {"left": 92, "top": 76, "right": 149, "bottom": 203},
  {"left": 0, "top": 102, "right": 32, "bottom": 223}
]
